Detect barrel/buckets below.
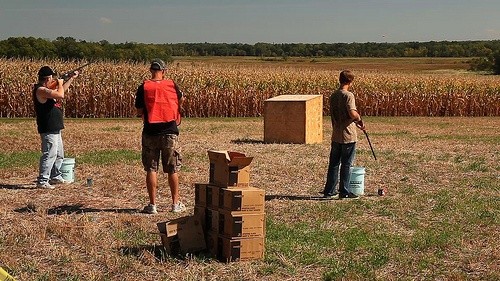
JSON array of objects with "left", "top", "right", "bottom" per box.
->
[
  {"left": 58, "top": 158, "right": 77, "bottom": 184},
  {"left": 349, "top": 167, "right": 365, "bottom": 195}
]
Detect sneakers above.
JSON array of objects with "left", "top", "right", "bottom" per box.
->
[
  {"left": 339, "top": 192, "right": 359, "bottom": 200},
  {"left": 37, "top": 181, "right": 55, "bottom": 189},
  {"left": 172, "top": 201, "right": 186, "bottom": 212},
  {"left": 324, "top": 193, "right": 340, "bottom": 200},
  {"left": 144, "top": 204, "right": 157, "bottom": 213},
  {"left": 48, "top": 175, "right": 67, "bottom": 184}
]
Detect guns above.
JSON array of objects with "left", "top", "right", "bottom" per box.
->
[
  {"left": 354, "top": 115, "right": 377, "bottom": 160},
  {"left": 46, "top": 62, "right": 91, "bottom": 90}
]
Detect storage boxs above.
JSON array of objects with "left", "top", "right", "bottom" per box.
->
[
  {"left": 264, "top": 95, "right": 323, "bottom": 144},
  {"left": 208, "top": 151, "right": 254, "bottom": 187},
  {"left": 194, "top": 183, "right": 265, "bottom": 260},
  {"left": 149, "top": 216, "right": 207, "bottom": 256}
]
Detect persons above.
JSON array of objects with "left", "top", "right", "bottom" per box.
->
[
  {"left": 135, "top": 58, "right": 186, "bottom": 214},
  {"left": 322, "top": 70, "right": 367, "bottom": 200},
  {"left": 32, "top": 66, "right": 79, "bottom": 190}
]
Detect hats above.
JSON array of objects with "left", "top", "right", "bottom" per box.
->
[
  {"left": 151, "top": 58, "right": 166, "bottom": 70},
  {"left": 38, "top": 66, "right": 55, "bottom": 75}
]
